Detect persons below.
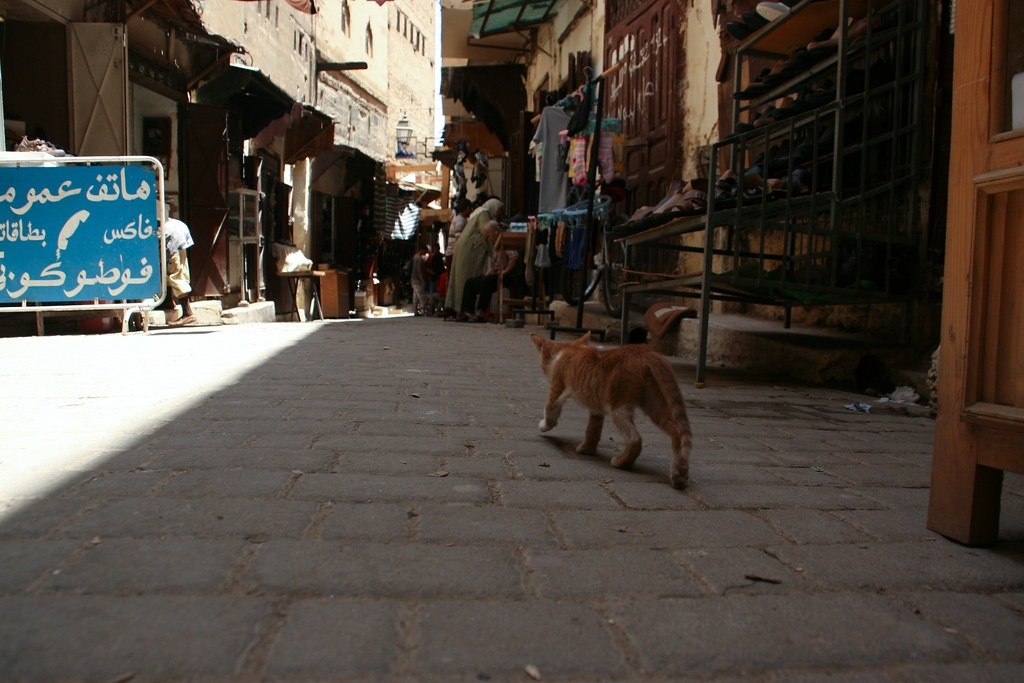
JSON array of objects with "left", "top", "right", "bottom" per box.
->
[
  {"left": 444, "top": 198, "right": 504, "bottom": 322},
  {"left": 410, "top": 242, "right": 446, "bottom": 317},
  {"left": 157, "top": 217, "right": 198, "bottom": 326},
  {"left": 457, "top": 220, "right": 519, "bottom": 323}
]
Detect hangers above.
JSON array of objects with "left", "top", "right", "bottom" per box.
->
[{"left": 553, "top": 65, "right": 592, "bottom": 119}]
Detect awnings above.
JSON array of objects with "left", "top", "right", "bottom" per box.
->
[
  {"left": 398, "top": 182, "right": 441, "bottom": 205},
  {"left": 126, "top": 0, "right": 384, "bottom": 189},
  {"left": 466, "top": 0, "right": 558, "bottom": 53}
]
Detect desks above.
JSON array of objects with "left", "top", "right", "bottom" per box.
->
[{"left": 277, "top": 271, "right": 327, "bottom": 322}]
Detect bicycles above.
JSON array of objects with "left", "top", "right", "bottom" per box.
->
[{"left": 560, "top": 189, "right": 632, "bottom": 318}]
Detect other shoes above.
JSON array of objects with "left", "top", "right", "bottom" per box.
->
[
  {"left": 426, "top": 312, "right": 433, "bottom": 316},
  {"left": 414, "top": 313, "right": 421, "bottom": 316},
  {"left": 167, "top": 313, "right": 196, "bottom": 326}
]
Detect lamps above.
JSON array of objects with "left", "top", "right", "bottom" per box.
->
[{"left": 394, "top": 112, "right": 414, "bottom": 146}]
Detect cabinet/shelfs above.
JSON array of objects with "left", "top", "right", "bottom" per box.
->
[{"left": 612, "top": 1, "right": 925, "bottom": 387}]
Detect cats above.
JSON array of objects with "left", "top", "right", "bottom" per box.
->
[{"left": 529, "top": 331, "right": 694, "bottom": 488}]
